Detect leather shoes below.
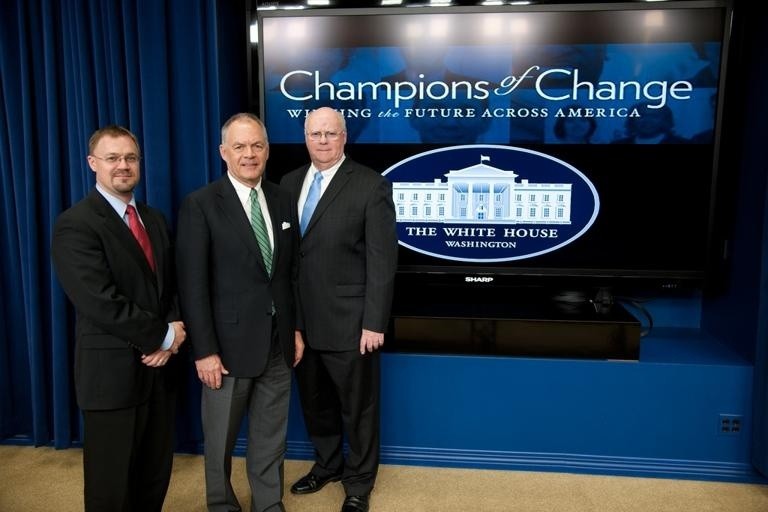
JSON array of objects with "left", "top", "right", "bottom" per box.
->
[
  {"left": 342, "top": 494, "right": 368, "bottom": 512},
  {"left": 291, "top": 472, "right": 342, "bottom": 494}
]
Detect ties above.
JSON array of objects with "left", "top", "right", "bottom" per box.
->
[
  {"left": 300, "top": 170, "right": 323, "bottom": 238},
  {"left": 250, "top": 187, "right": 276, "bottom": 318},
  {"left": 126, "top": 205, "right": 156, "bottom": 275}
]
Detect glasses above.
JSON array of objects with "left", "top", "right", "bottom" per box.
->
[
  {"left": 93, "top": 155, "right": 138, "bottom": 165},
  {"left": 306, "top": 130, "right": 344, "bottom": 139}
]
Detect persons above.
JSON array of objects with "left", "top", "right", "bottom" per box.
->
[
  {"left": 268, "top": 42, "right": 721, "bottom": 146},
  {"left": 281, "top": 106, "right": 399, "bottom": 512},
  {"left": 53, "top": 124, "right": 188, "bottom": 512},
  {"left": 176, "top": 109, "right": 303, "bottom": 512}
]
